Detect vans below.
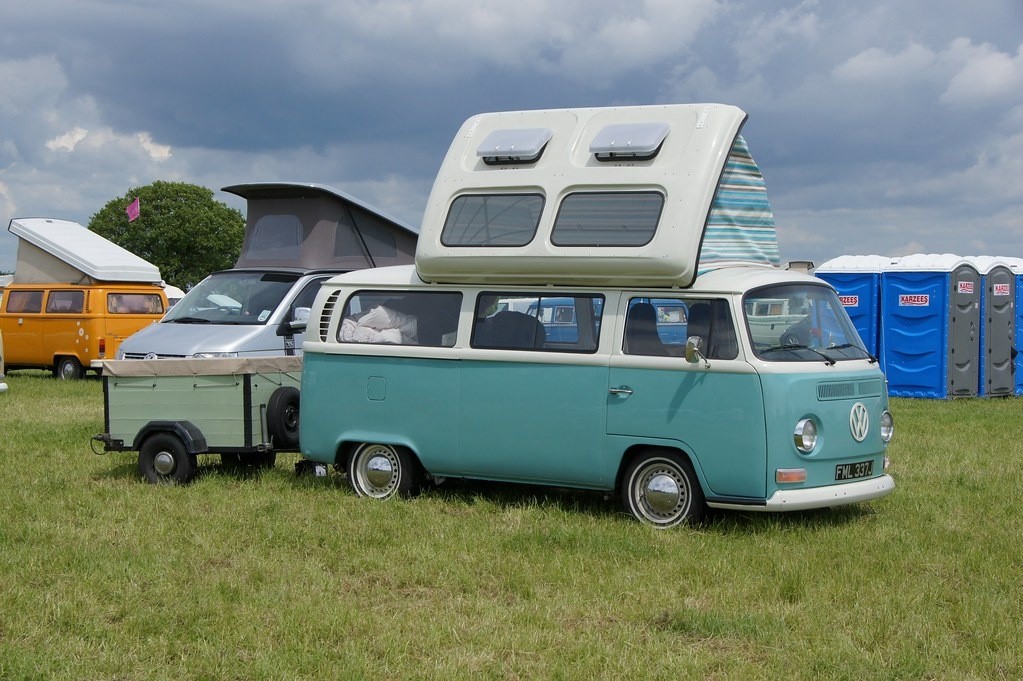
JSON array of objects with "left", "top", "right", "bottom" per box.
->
[
  {"left": 0, "top": 218, "right": 169, "bottom": 380},
  {"left": 114, "top": 183, "right": 419, "bottom": 363},
  {"left": 489, "top": 297, "right": 554, "bottom": 324},
  {"left": 297, "top": 104, "right": 896, "bottom": 532},
  {"left": 524, "top": 299, "right": 690, "bottom": 351}
]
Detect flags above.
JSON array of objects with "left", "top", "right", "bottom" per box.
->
[{"left": 127, "top": 198, "right": 140, "bottom": 222}]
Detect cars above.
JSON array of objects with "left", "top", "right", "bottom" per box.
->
[{"left": 778, "top": 314, "right": 812, "bottom": 347}]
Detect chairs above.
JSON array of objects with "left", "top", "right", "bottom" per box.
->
[
  {"left": 684, "top": 303, "right": 725, "bottom": 361},
  {"left": 491, "top": 311, "right": 547, "bottom": 349},
  {"left": 626, "top": 302, "right": 673, "bottom": 356}
]
